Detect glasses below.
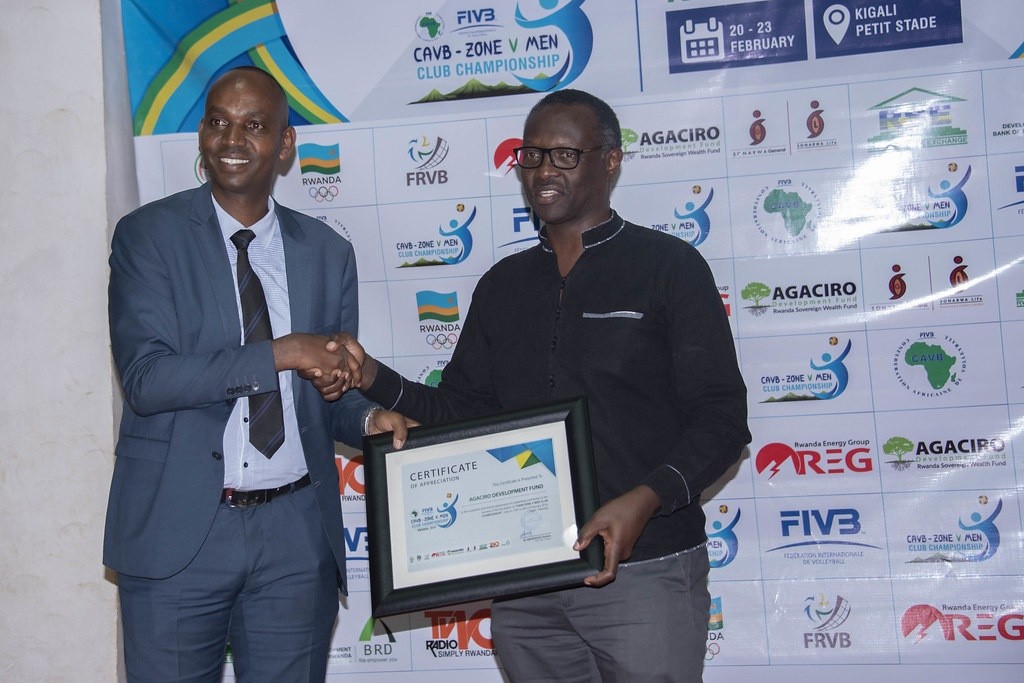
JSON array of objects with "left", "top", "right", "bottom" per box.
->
[{"left": 512, "top": 146, "right": 602, "bottom": 170}]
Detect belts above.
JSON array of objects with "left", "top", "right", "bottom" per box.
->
[
  {"left": 219, "top": 472, "right": 311, "bottom": 508},
  {"left": 229, "top": 229, "right": 286, "bottom": 460}
]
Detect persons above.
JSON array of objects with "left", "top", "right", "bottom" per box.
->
[
  {"left": 298, "top": 90, "right": 752, "bottom": 683},
  {"left": 100, "top": 64, "right": 425, "bottom": 683}
]
